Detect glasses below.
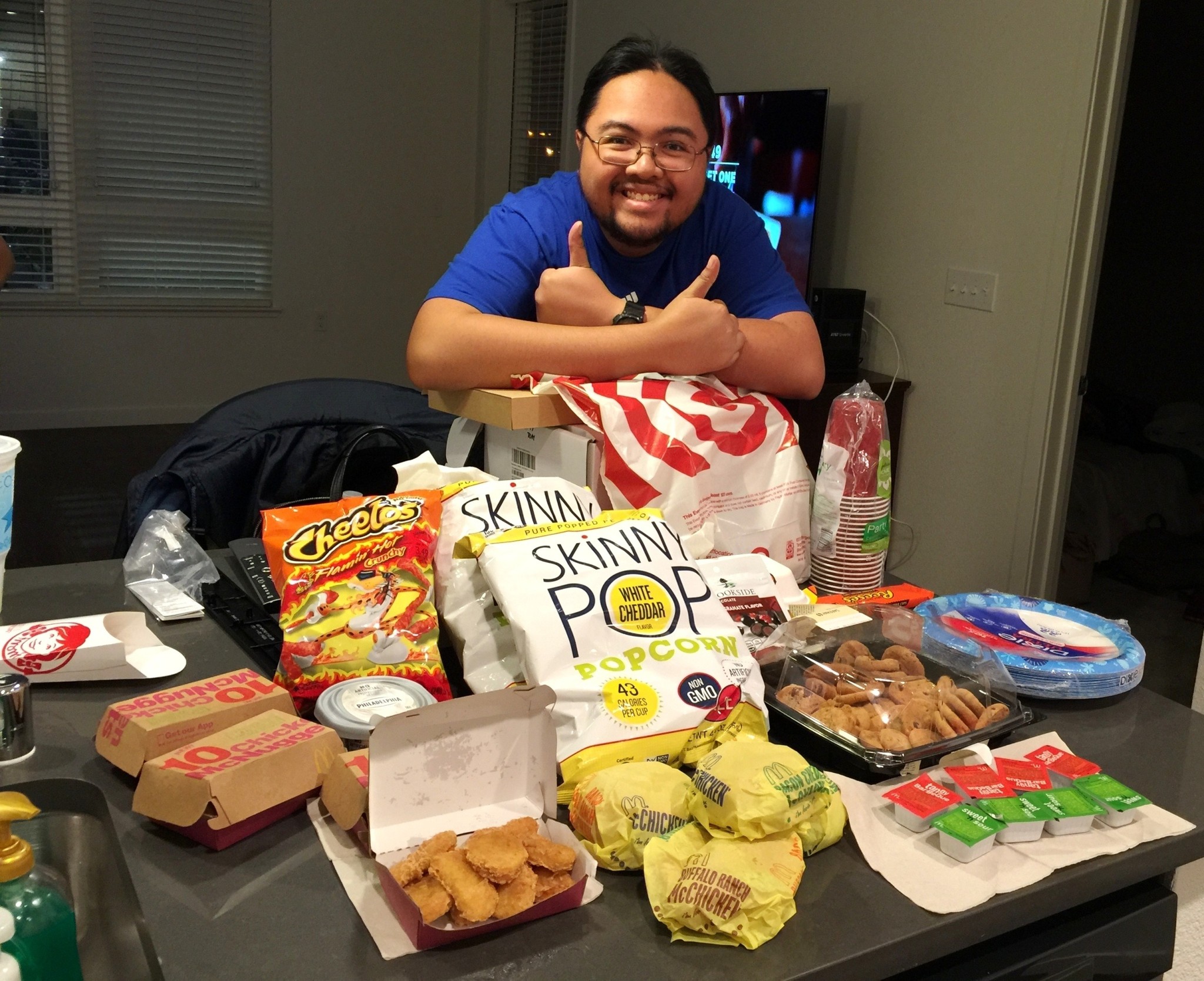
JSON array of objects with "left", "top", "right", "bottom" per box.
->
[{"left": 580, "top": 129, "right": 711, "bottom": 171}]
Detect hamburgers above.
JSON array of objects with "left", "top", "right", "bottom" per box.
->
[{"left": 567, "top": 738, "right": 851, "bottom": 944}]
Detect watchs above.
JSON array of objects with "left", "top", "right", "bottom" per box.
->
[{"left": 611, "top": 300, "right": 645, "bottom": 325}]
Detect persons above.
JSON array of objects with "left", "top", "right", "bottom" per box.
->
[{"left": 406, "top": 34, "right": 826, "bottom": 399}]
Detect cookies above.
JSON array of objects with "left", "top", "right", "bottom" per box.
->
[{"left": 771, "top": 640, "right": 1009, "bottom": 755}]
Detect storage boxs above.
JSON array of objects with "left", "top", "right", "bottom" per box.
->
[
  {"left": 428, "top": 362, "right": 584, "bottom": 430},
  {"left": 369, "top": 681, "right": 589, "bottom": 953},
  {"left": 126, "top": 707, "right": 348, "bottom": 856},
  {"left": 483, "top": 423, "right": 611, "bottom": 513},
  {"left": 92, "top": 667, "right": 296, "bottom": 776},
  {"left": 320, "top": 743, "right": 375, "bottom": 855}
]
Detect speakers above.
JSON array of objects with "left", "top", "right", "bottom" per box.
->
[{"left": 812, "top": 287, "right": 866, "bottom": 383}]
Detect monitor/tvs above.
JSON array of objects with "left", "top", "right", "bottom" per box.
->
[{"left": 703, "top": 89, "right": 829, "bottom": 302}]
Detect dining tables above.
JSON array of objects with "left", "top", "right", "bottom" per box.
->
[{"left": 7, "top": 538, "right": 1201, "bottom": 981}]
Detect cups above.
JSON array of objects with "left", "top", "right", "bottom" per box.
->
[{"left": 808, "top": 393, "right": 894, "bottom": 598}]
